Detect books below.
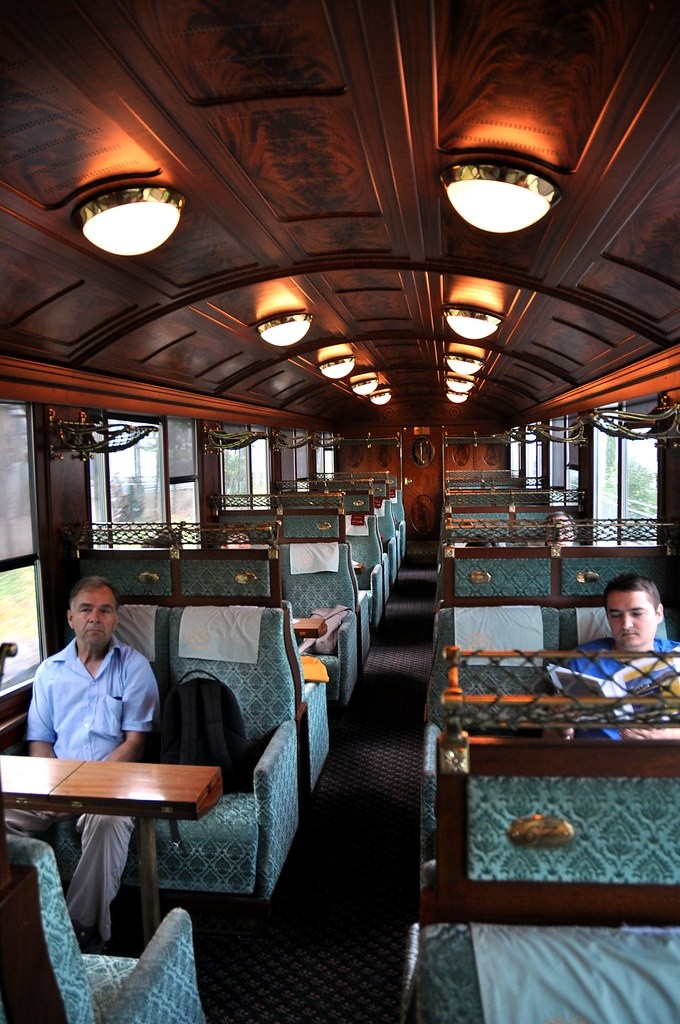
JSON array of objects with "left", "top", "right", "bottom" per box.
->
[{"left": 547, "top": 645, "right": 680, "bottom": 723}]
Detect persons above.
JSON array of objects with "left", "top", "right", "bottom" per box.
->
[
  {"left": 535, "top": 573, "right": 680, "bottom": 741},
  {"left": 543, "top": 511, "right": 575, "bottom": 547},
  {"left": 219, "top": 524, "right": 251, "bottom": 550},
  {"left": 1, "top": 575, "right": 162, "bottom": 952}
]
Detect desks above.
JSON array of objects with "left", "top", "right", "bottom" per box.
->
[
  {"left": 353, "top": 563, "right": 365, "bottom": 574},
  {"left": 0, "top": 755, "right": 223, "bottom": 951},
  {"left": 293, "top": 618, "right": 328, "bottom": 638}
]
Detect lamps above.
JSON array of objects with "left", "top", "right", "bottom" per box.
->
[
  {"left": 369, "top": 390, "right": 392, "bottom": 406},
  {"left": 445, "top": 376, "right": 474, "bottom": 392},
  {"left": 351, "top": 379, "right": 379, "bottom": 395},
  {"left": 445, "top": 354, "right": 485, "bottom": 375},
  {"left": 439, "top": 164, "right": 563, "bottom": 234},
  {"left": 442, "top": 309, "right": 504, "bottom": 340},
  {"left": 255, "top": 313, "right": 314, "bottom": 346},
  {"left": 319, "top": 357, "right": 355, "bottom": 379},
  {"left": 446, "top": 390, "right": 468, "bottom": 403},
  {"left": 69, "top": 187, "right": 186, "bottom": 256}
]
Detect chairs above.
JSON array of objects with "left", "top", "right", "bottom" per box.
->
[
  {"left": 400, "top": 478, "right": 680, "bottom": 1024},
  {"left": 0, "top": 473, "right": 407, "bottom": 927},
  {"left": 0, "top": 834, "right": 208, "bottom": 1024}
]
